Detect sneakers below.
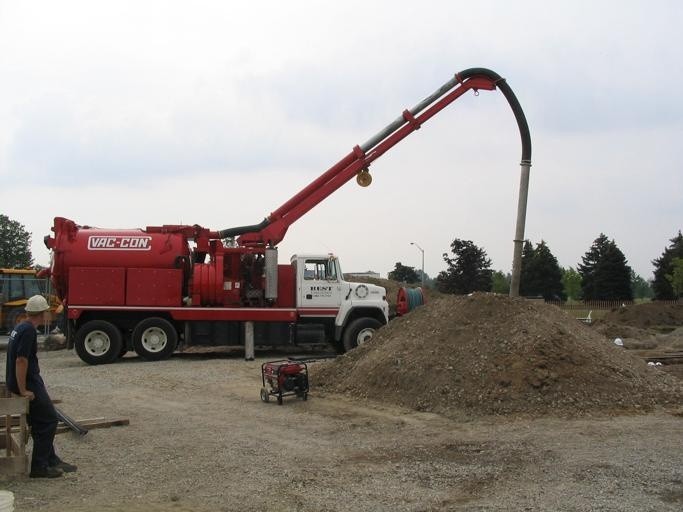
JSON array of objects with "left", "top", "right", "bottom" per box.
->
[{"left": 29, "top": 461, "right": 76, "bottom": 478}]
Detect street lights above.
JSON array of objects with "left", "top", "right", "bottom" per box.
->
[{"left": 410, "top": 242, "right": 424, "bottom": 288}]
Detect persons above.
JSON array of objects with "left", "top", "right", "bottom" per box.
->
[{"left": 5, "top": 292, "right": 78, "bottom": 479}]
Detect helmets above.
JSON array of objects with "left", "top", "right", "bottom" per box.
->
[{"left": 24, "top": 295, "right": 49, "bottom": 313}]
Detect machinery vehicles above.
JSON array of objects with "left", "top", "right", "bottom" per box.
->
[
  {"left": 0, "top": 268, "right": 63, "bottom": 335},
  {"left": 44, "top": 65, "right": 532, "bottom": 366}
]
